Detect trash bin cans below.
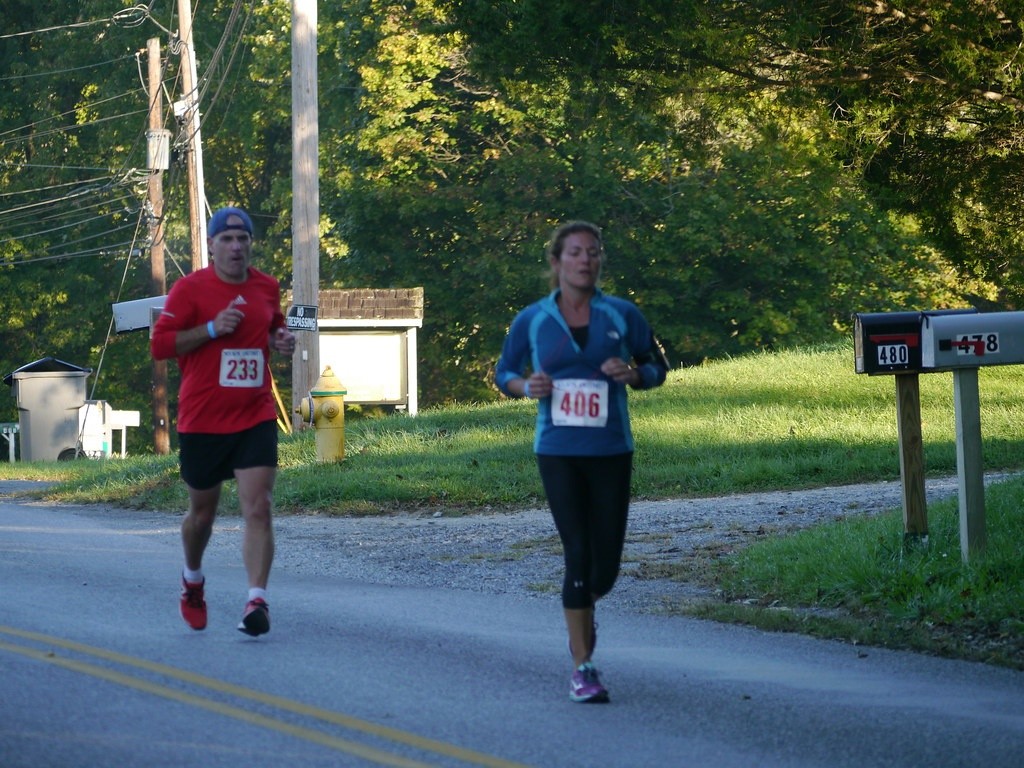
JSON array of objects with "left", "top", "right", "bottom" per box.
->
[{"left": 3, "top": 357, "right": 93, "bottom": 465}]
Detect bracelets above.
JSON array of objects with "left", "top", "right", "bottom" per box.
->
[
  {"left": 207, "top": 320, "right": 217, "bottom": 338},
  {"left": 524, "top": 380, "right": 532, "bottom": 399}
]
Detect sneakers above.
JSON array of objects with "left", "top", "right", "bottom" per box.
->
[
  {"left": 569, "top": 603, "right": 598, "bottom": 663},
  {"left": 180, "top": 570, "right": 208, "bottom": 630},
  {"left": 237, "top": 598, "right": 271, "bottom": 636},
  {"left": 569, "top": 667, "right": 608, "bottom": 704}
]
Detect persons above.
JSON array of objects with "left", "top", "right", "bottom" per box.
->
[
  {"left": 151, "top": 208, "right": 299, "bottom": 637},
  {"left": 494, "top": 220, "right": 670, "bottom": 703}
]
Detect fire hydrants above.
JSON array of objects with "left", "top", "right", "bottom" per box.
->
[{"left": 295, "top": 365, "right": 348, "bottom": 466}]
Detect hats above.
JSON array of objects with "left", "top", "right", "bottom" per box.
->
[{"left": 208, "top": 207, "right": 251, "bottom": 237}]
